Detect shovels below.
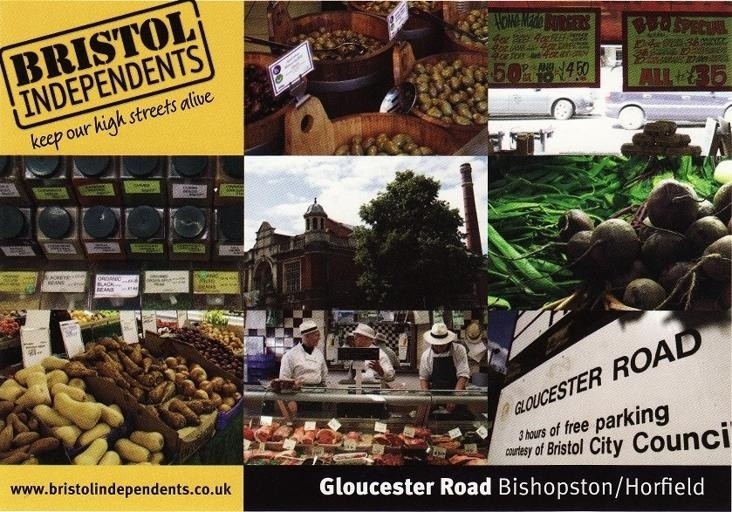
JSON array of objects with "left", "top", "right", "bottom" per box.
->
[{"left": 243, "top": 35, "right": 368, "bottom": 57}]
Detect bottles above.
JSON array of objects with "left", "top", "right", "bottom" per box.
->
[
  {"left": 516, "top": 130, "right": 533, "bottom": 155},
  {"left": 264, "top": 346, "right": 269, "bottom": 355}
]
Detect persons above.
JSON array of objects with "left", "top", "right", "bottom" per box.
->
[
  {"left": 338, "top": 323, "right": 397, "bottom": 420},
  {"left": 341, "top": 331, "right": 353, "bottom": 369},
  {"left": 418, "top": 322, "right": 473, "bottom": 422},
  {"left": 278, "top": 321, "right": 334, "bottom": 420},
  {"left": 373, "top": 333, "right": 401, "bottom": 370}
]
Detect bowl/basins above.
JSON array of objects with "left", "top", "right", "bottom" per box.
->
[{"left": 257, "top": 379, "right": 272, "bottom": 389}]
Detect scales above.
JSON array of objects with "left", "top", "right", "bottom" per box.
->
[{"left": 336, "top": 347, "right": 389, "bottom": 419}]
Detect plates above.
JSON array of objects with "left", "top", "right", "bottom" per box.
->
[{"left": 333, "top": 452, "right": 367, "bottom": 463}]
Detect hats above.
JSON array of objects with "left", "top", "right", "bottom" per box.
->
[
  {"left": 465, "top": 322, "right": 483, "bottom": 344},
  {"left": 352, "top": 323, "right": 377, "bottom": 340},
  {"left": 374, "top": 332, "right": 387, "bottom": 343},
  {"left": 293, "top": 320, "right": 325, "bottom": 338},
  {"left": 423, "top": 322, "right": 456, "bottom": 346}
]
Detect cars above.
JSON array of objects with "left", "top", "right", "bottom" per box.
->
[
  {"left": 488, "top": 85, "right": 595, "bottom": 122},
  {"left": 605, "top": 65, "right": 732, "bottom": 135}
]
